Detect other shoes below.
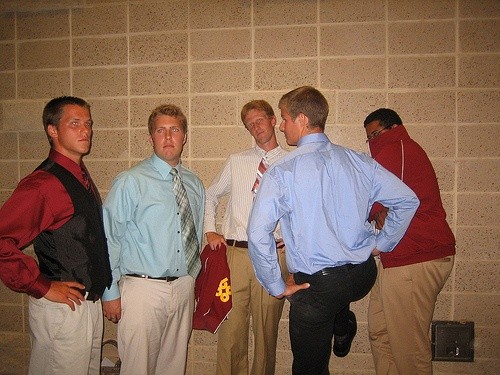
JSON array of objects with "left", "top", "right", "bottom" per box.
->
[{"left": 332, "top": 310, "right": 357, "bottom": 357}]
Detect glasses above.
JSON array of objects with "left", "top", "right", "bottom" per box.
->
[{"left": 366, "top": 126, "right": 389, "bottom": 143}]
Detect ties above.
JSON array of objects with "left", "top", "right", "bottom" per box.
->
[
  {"left": 252, "top": 154, "right": 269, "bottom": 194},
  {"left": 81, "top": 171, "right": 95, "bottom": 198},
  {"left": 169, "top": 168, "right": 202, "bottom": 280}
]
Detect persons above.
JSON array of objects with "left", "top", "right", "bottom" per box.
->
[
  {"left": 101, "top": 104, "right": 206, "bottom": 375},
  {"left": 245, "top": 85, "right": 421, "bottom": 375},
  {"left": 0, "top": 95, "right": 114, "bottom": 375},
  {"left": 204, "top": 99, "right": 292, "bottom": 375},
  {"left": 363, "top": 108, "right": 456, "bottom": 375}
]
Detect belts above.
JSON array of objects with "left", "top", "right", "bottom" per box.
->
[
  {"left": 125, "top": 273, "right": 179, "bottom": 282},
  {"left": 321, "top": 264, "right": 364, "bottom": 275},
  {"left": 225, "top": 239, "right": 285, "bottom": 249},
  {"left": 77, "top": 290, "right": 101, "bottom": 303}
]
